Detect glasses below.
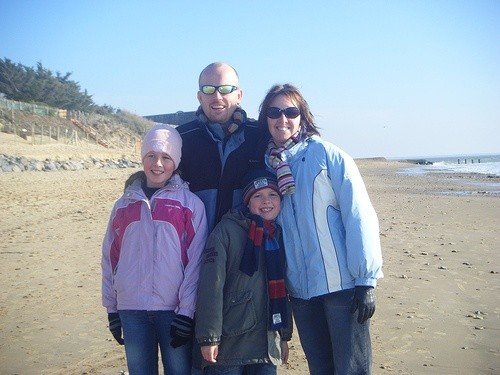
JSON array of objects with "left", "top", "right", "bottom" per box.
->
[
  {"left": 264, "top": 107, "right": 303, "bottom": 120},
  {"left": 198, "top": 85, "right": 238, "bottom": 94}
]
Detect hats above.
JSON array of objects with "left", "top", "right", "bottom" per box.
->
[
  {"left": 140, "top": 125, "right": 182, "bottom": 170},
  {"left": 241, "top": 165, "right": 285, "bottom": 207}
]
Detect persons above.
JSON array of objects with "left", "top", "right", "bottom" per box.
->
[
  {"left": 123, "top": 61, "right": 266, "bottom": 236},
  {"left": 101, "top": 124, "right": 203, "bottom": 375},
  {"left": 191, "top": 161, "right": 294, "bottom": 375},
  {"left": 233, "top": 83, "right": 384, "bottom": 374}
]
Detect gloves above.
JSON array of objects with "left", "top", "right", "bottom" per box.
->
[
  {"left": 168, "top": 314, "right": 194, "bottom": 348},
  {"left": 351, "top": 286, "right": 376, "bottom": 322},
  {"left": 107, "top": 311, "right": 125, "bottom": 345}
]
